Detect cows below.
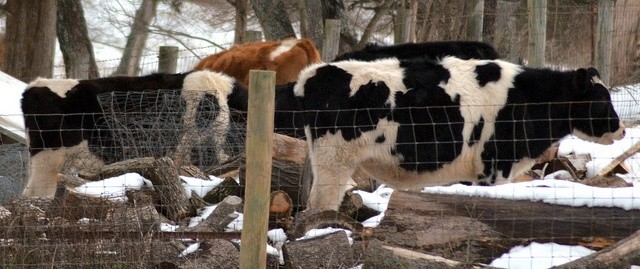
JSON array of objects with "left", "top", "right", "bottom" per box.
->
[
  {"left": 20, "top": 69, "right": 300, "bottom": 202},
  {"left": 330, "top": 39, "right": 503, "bottom": 62},
  {"left": 191, "top": 37, "right": 323, "bottom": 87},
  {"left": 291, "top": 56, "right": 627, "bottom": 216}
]
